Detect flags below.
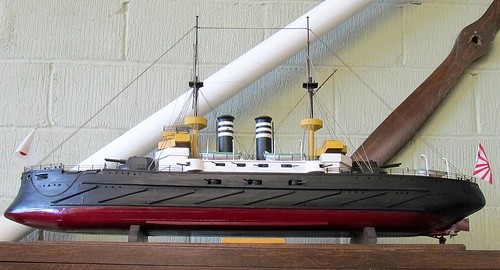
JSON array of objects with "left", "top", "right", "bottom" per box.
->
[
  {"left": 14, "top": 128, "right": 41, "bottom": 162},
  {"left": 472, "top": 143, "right": 493, "bottom": 185}
]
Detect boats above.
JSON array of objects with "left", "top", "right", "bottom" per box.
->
[{"left": 3, "top": 13, "right": 486, "bottom": 239}]
